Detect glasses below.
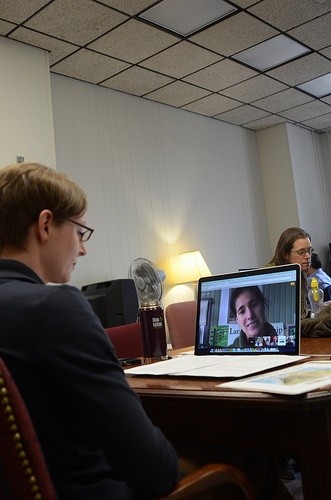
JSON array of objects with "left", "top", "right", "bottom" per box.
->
[
  {"left": 32, "top": 208, "right": 93, "bottom": 243},
  {"left": 290, "top": 247, "right": 314, "bottom": 256}
]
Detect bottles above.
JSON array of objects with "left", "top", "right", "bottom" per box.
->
[
  {"left": 308, "top": 278, "right": 324, "bottom": 319},
  {"left": 138, "top": 301, "right": 167, "bottom": 364}
]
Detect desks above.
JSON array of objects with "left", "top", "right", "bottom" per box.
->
[{"left": 122, "top": 338, "right": 331, "bottom": 500}]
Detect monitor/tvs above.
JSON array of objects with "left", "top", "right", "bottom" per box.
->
[{"left": 80, "top": 279, "right": 140, "bottom": 329}]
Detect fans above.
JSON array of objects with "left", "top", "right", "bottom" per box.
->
[{"left": 128, "top": 257, "right": 166, "bottom": 301}]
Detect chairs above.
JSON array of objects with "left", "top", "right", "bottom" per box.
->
[
  {"left": 1, "top": 363, "right": 256, "bottom": 500},
  {"left": 171, "top": 250, "right": 212, "bottom": 299},
  {"left": 166, "top": 300, "right": 197, "bottom": 349}
]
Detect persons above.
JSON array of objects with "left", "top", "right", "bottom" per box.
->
[
  {"left": 262, "top": 227, "right": 313, "bottom": 318},
  {"left": 0, "top": 161, "right": 293, "bottom": 500},
  {"left": 228, "top": 285, "right": 294, "bottom": 347},
  {"left": 306, "top": 253, "right": 331, "bottom": 297}
]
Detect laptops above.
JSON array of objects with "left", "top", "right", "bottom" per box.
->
[{"left": 123, "top": 263, "right": 302, "bottom": 379}]
